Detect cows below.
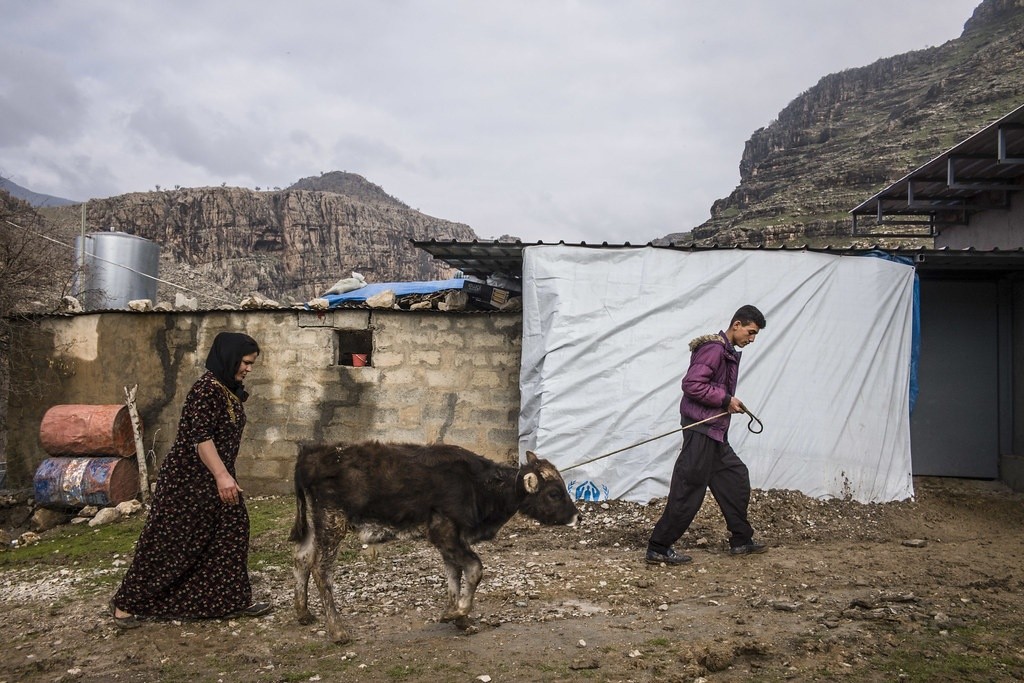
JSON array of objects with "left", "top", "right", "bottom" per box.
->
[{"left": 283, "top": 437, "right": 584, "bottom": 645}]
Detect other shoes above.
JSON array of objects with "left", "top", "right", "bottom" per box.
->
[
  {"left": 731, "top": 542, "right": 768, "bottom": 554},
  {"left": 108, "top": 599, "right": 140, "bottom": 629},
  {"left": 645, "top": 546, "right": 692, "bottom": 564},
  {"left": 235, "top": 603, "right": 270, "bottom": 616}
]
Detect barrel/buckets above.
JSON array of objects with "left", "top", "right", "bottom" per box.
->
[
  {"left": 40, "top": 404, "right": 142, "bottom": 456},
  {"left": 33, "top": 457, "right": 141, "bottom": 513},
  {"left": 352, "top": 354, "right": 368, "bottom": 367}
]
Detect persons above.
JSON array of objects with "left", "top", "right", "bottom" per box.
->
[
  {"left": 109, "top": 334, "right": 273, "bottom": 628},
  {"left": 646, "top": 305, "right": 766, "bottom": 564}
]
